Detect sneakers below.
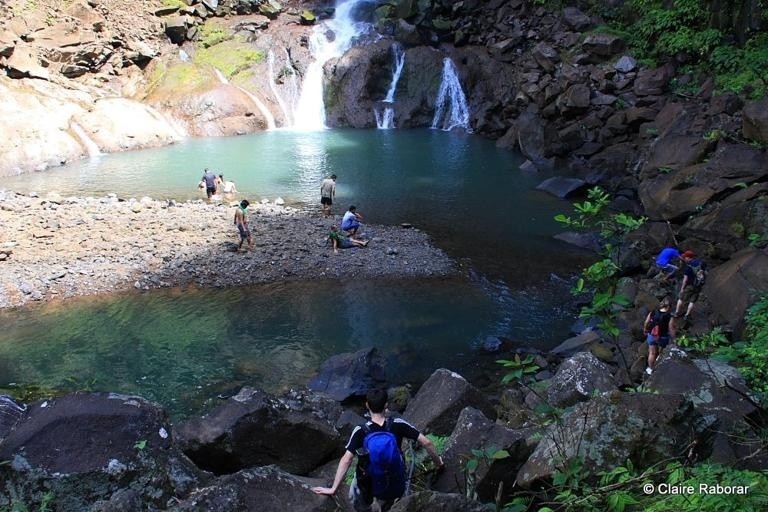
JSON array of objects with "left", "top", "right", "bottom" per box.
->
[
  {"left": 682, "top": 314, "right": 688, "bottom": 321},
  {"left": 646, "top": 366, "right": 653, "bottom": 375},
  {"left": 670, "top": 312, "right": 681, "bottom": 318}
]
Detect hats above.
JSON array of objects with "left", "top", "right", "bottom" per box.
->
[{"left": 682, "top": 251, "right": 694, "bottom": 258}]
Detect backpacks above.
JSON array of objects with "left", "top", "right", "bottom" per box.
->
[
  {"left": 645, "top": 310, "right": 669, "bottom": 335},
  {"left": 687, "top": 261, "right": 705, "bottom": 288},
  {"left": 475, "top": 437, "right": 530, "bottom": 504},
  {"left": 358, "top": 416, "right": 406, "bottom": 501}
]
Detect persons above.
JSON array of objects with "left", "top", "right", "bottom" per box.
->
[
  {"left": 670, "top": 250, "right": 708, "bottom": 321},
  {"left": 199, "top": 168, "right": 238, "bottom": 201},
  {"left": 234, "top": 200, "right": 252, "bottom": 250},
  {"left": 354, "top": 210, "right": 364, "bottom": 221},
  {"left": 642, "top": 296, "right": 676, "bottom": 375},
  {"left": 339, "top": 206, "right": 360, "bottom": 238},
  {"left": 321, "top": 225, "right": 369, "bottom": 255},
  {"left": 319, "top": 174, "right": 337, "bottom": 218},
  {"left": 309, "top": 386, "right": 442, "bottom": 511}
]
